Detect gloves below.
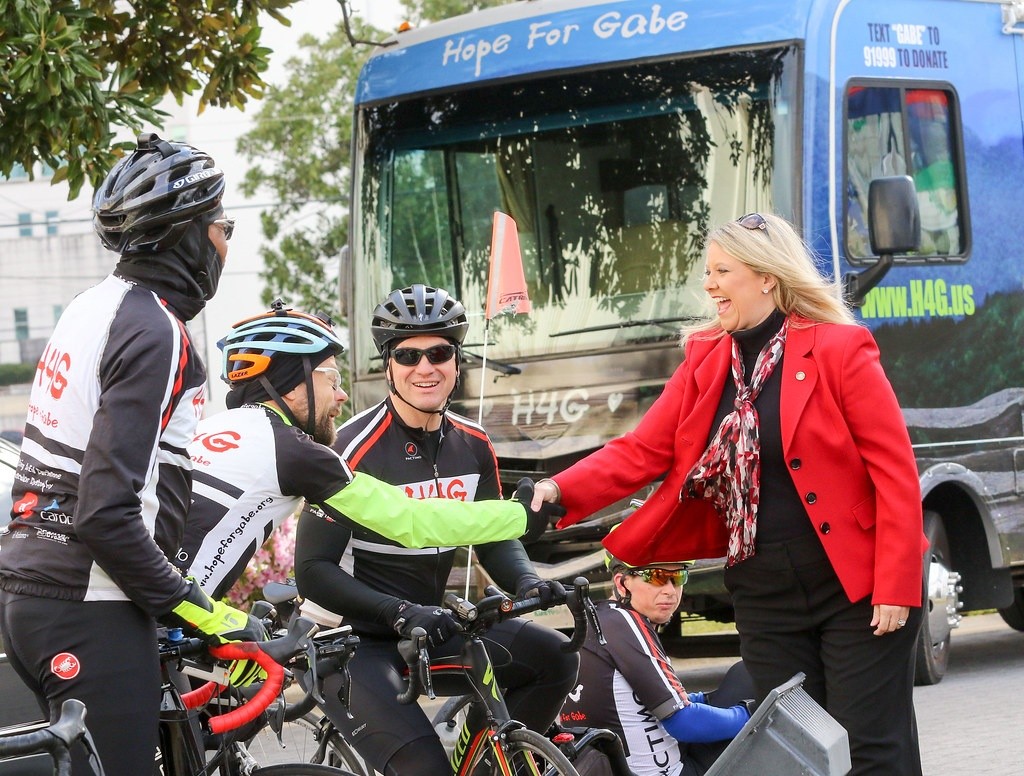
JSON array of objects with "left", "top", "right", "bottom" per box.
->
[
  {"left": 156, "top": 576, "right": 289, "bottom": 689},
  {"left": 703, "top": 689, "right": 724, "bottom": 708},
  {"left": 385, "top": 600, "right": 466, "bottom": 652},
  {"left": 516, "top": 574, "right": 575, "bottom": 611},
  {"left": 738, "top": 698, "right": 758, "bottom": 717},
  {"left": 514, "top": 477, "right": 566, "bottom": 545}
]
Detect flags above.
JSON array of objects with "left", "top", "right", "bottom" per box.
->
[{"left": 484, "top": 212, "right": 530, "bottom": 320}]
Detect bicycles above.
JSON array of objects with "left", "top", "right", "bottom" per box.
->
[{"left": 0, "top": 577, "right": 607, "bottom": 775}]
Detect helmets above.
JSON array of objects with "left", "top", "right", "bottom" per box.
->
[
  {"left": 605, "top": 498, "right": 696, "bottom": 574},
  {"left": 90, "top": 132, "right": 225, "bottom": 255},
  {"left": 370, "top": 284, "right": 469, "bottom": 370},
  {"left": 216, "top": 298, "right": 345, "bottom": 406}
]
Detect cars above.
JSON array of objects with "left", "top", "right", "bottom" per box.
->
[{"left": 0, "top": 439, "right": 58, "bottom": 776}]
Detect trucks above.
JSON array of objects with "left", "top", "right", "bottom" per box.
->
[{"left": 341, "top": 1, "right": 1024, "bottom": 684}]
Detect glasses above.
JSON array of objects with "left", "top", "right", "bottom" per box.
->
[
  {"left": 207, "top": 219, "right": 235, "bottom": 241},
  {"left": 618, "top": 568, "right": 689, "bottom": 588},
  {"left": 390, "top": 344, "right": 457, "bottom": 366},
  {"left": 735, "top": 213, "right": 773, "bottom": 243},
  {"left": 313, "top": 367, "right": 341, "bottom": 391}
]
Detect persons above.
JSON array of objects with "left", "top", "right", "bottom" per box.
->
[
  {"left": 509, "top": 212, "right": 931, "bottom": 776},
  {"left": 294, "top": 284, "right": 581, "bottom": 776},
  {"left": 559, "top": 522, "right": 758, "bottom": 776},
  {"left": 155, "top": 299, "right": 569, "bottom": 638},
  {"left": 0, "top": 132, "right": 268, "bottom": 776}
]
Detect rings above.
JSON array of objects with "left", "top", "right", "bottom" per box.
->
[{"left": 898, "top": 620, "right": 906, "bottom": 626}]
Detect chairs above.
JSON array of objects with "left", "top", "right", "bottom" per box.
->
[{"left": 593, "top": 217, "right": 695, "bottom": 295}]
